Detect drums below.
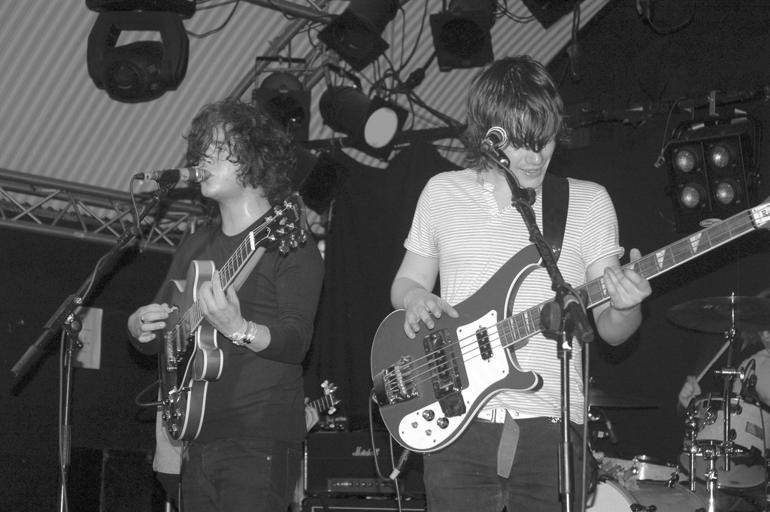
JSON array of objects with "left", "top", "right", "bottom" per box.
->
[
  {"left": 585, "top": 478, "right": 707, "bottom": 512},
  {"left": 681, "top": 394, "right": 769, "bottom": 489}
]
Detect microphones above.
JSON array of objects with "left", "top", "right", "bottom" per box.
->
[
  {"left": 480, "top": 126, "right": 510, "bottom": 153},
  {"left": 138, "top": 165, "right": 210, "bottom": 183}
]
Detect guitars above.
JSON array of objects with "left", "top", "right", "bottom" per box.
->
[
  {"left": 372, "top": 197, "right": 770, "bottom": 452},
  {"left": 160, "top": 192, "right": 308, "bottom": 438}
]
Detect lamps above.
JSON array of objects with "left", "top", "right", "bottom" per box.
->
[
  {"left": 651, "top": 80, "right": 768, "bottom": 222},
  {"left": 430, "top": 0, "right": 505, "bottom": 73},
  {"left": 299, "top": 76, "right": 415, "bottom": 165},
  {"left": 318, "top": 0, "right": 401, "bottom": 71},
  {"left": 86, "top": 0, "right": 199, "bottom": 103}
]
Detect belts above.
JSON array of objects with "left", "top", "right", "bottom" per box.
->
[{"left": 473, "top": 407, "right": 566, "bottom": 480}]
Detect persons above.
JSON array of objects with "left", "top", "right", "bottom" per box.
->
[
  {"left": 122, "top": 92, "right": 329, "bottom": 512},
  {"left": 388, "top": 54, "right": 654, "bottom": 512}
]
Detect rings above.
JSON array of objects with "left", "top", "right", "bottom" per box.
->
[{"left": 140, "top": 314, "right": 145, "bottom": 324}]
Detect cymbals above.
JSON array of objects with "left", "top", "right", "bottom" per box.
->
[
  {"left": 668, "top": 296, "right": 770, "bottom": 335},
  {"left": 586, "top": 387, "right": 662, "bottom": 408}
]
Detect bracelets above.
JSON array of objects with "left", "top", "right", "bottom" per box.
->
[
  {"left": 224, "top": 317, "right": 247, "bottom": 343},
  {"left": 232, "top": 320, "right": 257, "bottom": 347}
]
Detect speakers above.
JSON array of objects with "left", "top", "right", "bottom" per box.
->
[
  {"left": 304, "top": 430, "right": 426, "bottom": 497},
  {"left": 57, "top": 447, "right": 154, "bottom": 512},
  {"left": 302, "top": 497, "right": 427, "bottom": 512}
]
